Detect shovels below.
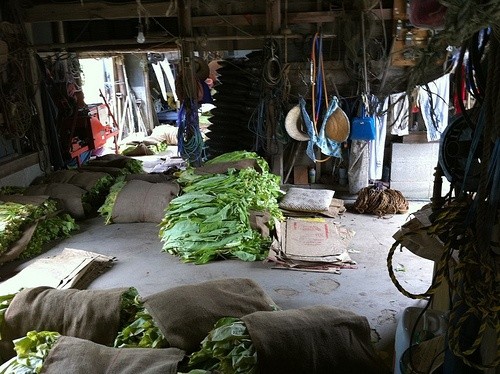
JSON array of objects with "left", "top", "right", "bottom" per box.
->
[{"left": 349, "top": 103, "right": 375, "bottom": 142}]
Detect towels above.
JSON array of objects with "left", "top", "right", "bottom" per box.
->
[{"left": 388, "top": 73, "right": 450, "bottom": 141}]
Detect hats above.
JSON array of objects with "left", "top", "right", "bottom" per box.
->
[
  {"left": 285, "top": 98, "right": 317, "bottom": 163},
  {"left": 316, "top": 96, "right": 350, "bottom": 157}
]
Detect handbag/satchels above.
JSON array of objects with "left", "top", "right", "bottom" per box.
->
[{"left": 350, "top": 103, "right": 375, "bottom": 140}]
